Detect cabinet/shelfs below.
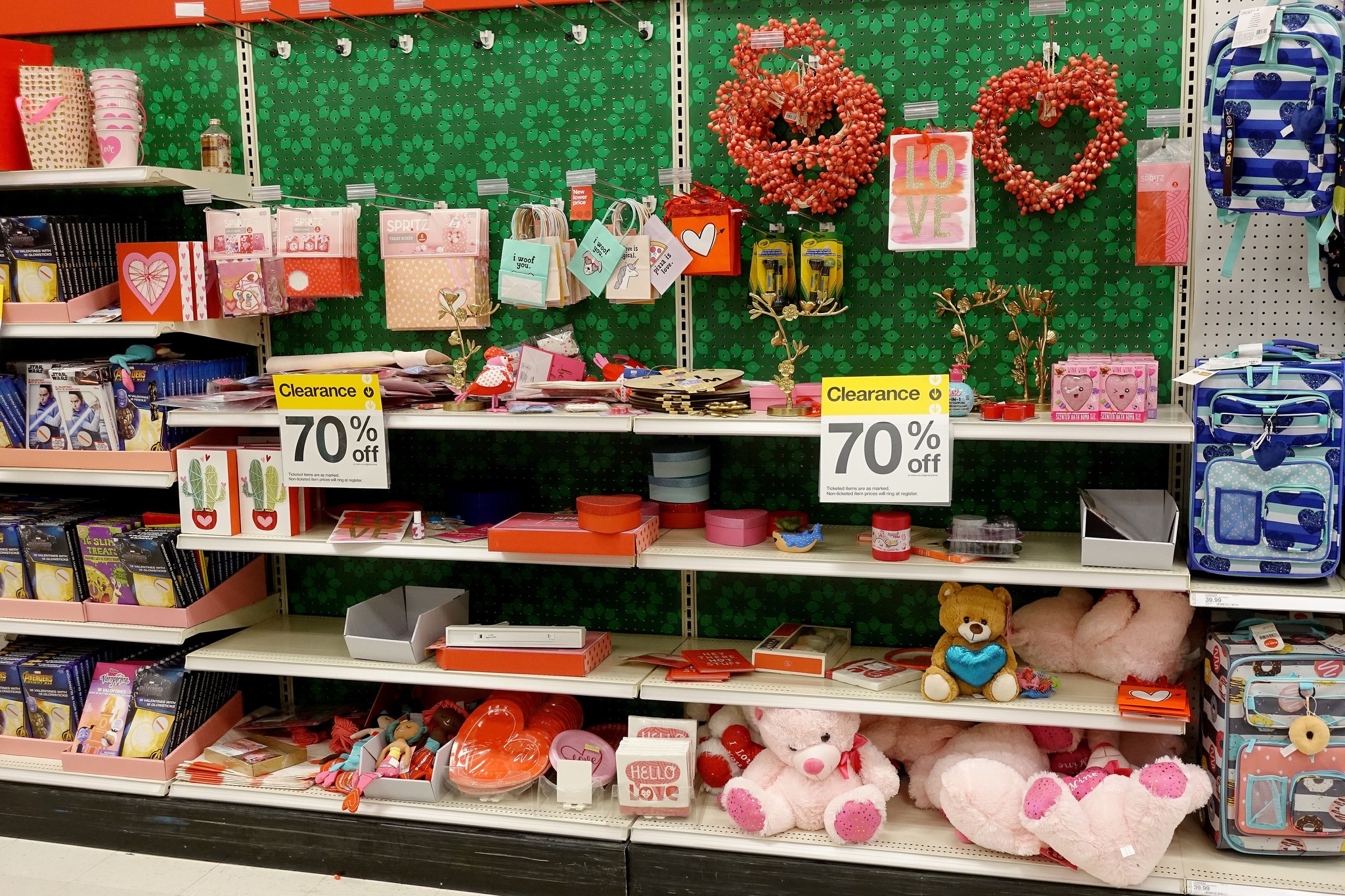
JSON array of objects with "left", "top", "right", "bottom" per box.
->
[
  {"left": 0, "top": 166, "right": 289, "bottom": 796},
  {"left": 634, "top": 405, "right": 1196, "bottom": 896},
  {"left": 164, "top": 394, "right": 689, "bottom": 846},
  {"left": 1189, "top": 567, "right": 1344, "bottom": 895}
]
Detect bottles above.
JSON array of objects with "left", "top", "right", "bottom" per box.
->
[{"left": 200, "top": 118, "right": 232, "bottom": 174}]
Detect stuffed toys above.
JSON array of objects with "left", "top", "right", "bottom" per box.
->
[
  {"left": 921, "top": 580, "right": 1019, "bottom": 702},
  {"left": 1000, "top": 585, "right": 1194, "bottom": 686},
  {"left": 683, "top": 704, "right": 1217, "bottom": 888}
]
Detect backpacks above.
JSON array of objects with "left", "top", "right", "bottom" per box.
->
[
  {"left": 1198, "top": 616, "right": 1345, "bottom": 859},
  {"left": 1186, "top": 339, "right": 1343, "bottom": 578},
  {"left": 1199, "top": 1, "right": 1345, "bottom": 217}
]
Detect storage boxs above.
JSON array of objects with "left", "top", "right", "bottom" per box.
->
[
  {"left": 1052, "top": 352, "right": 1160, "bottom": 423},
  {"left": 0, "top": 214, "right": 851, "bottom": 823},
  {"left": 1080, "top": 490, "right": 1181, "bottom": 571},
  {"left": 0, "top": 38, "right": 54, "bottom": 173}
]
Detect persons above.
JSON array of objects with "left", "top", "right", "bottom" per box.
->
[
  {"left": 399, "top": 699, "right": 470, "bottom": 781},
  {"left": 375, "top": 719, "right": 427, "bottom": 778},
  {"left": 344, "top": 710, "right": 396, "bottom": 771},
  {"left": 69, "top": 391, "right": 103, "bottom": 450},
  {"left": 36, "top": 384, "right": 62, "bottom": 449}
]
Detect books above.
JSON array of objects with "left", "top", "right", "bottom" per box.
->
[
  {"left": 665, "top": 649, "right": 757, "bottom": 683},
  {"left": 0, "top": 214, "right": 148, "bottom": 302}
]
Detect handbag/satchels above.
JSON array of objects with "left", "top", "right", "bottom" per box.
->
[{"left": 495, "top": 198, "right": 693, "bottom": 310}]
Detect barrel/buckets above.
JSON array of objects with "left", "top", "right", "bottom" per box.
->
[{"left": 86, "top": 68, "right": 148, "bottom": 168}]
